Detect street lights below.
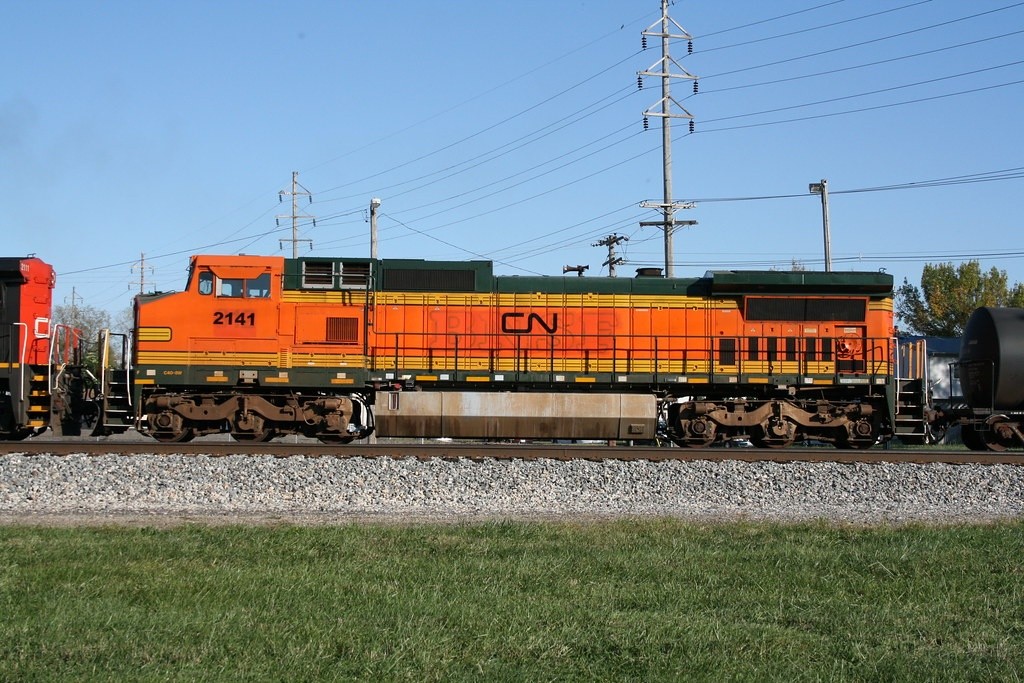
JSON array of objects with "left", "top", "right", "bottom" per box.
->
[
  {"left": 810, "top": 178, "right": 830, "bottom": 271},
  {"left": 369, "top": 198, "right": 381, "bottom": 259}
]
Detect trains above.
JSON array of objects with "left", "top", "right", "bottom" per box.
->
[{"left": 0, "top": 251, "right": 1024, "bottom": 452}]
